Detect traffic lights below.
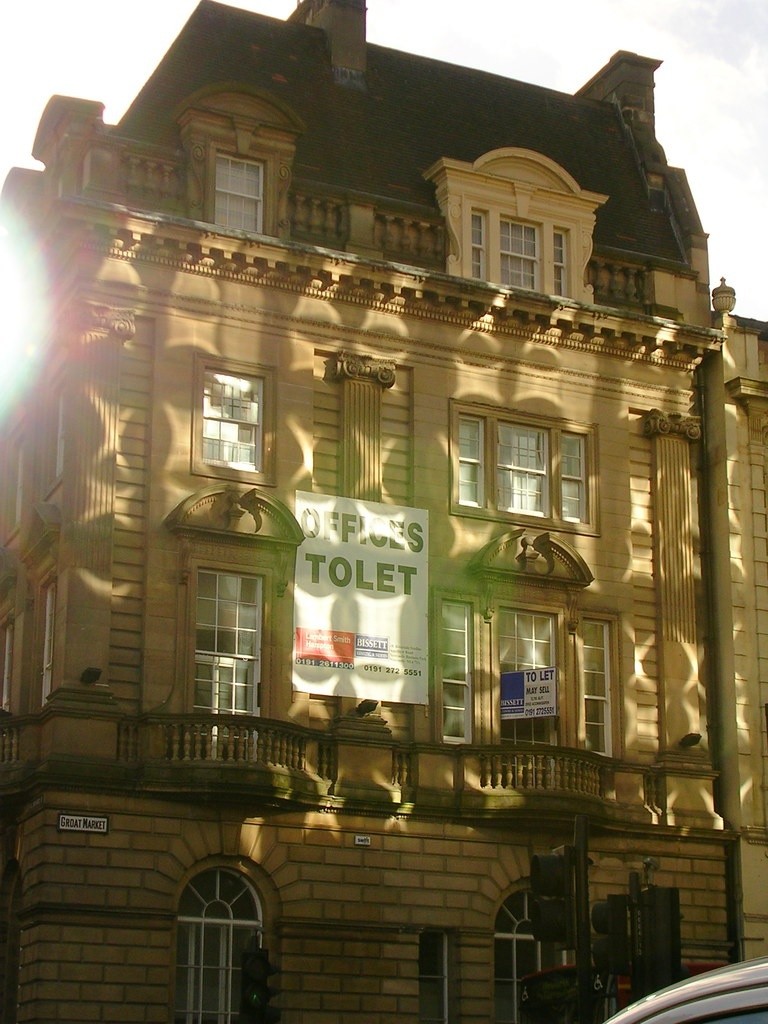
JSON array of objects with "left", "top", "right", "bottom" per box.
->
[
  {"left": 591, "top": 891, "right": 634, "bottom": 974},
  {"left": 527, "top": 843, "right": 576, "bottom": 952}
]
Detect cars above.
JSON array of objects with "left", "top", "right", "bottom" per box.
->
[{"left": 602, "top": 956, "right": 768, "bottom": 1024}]
